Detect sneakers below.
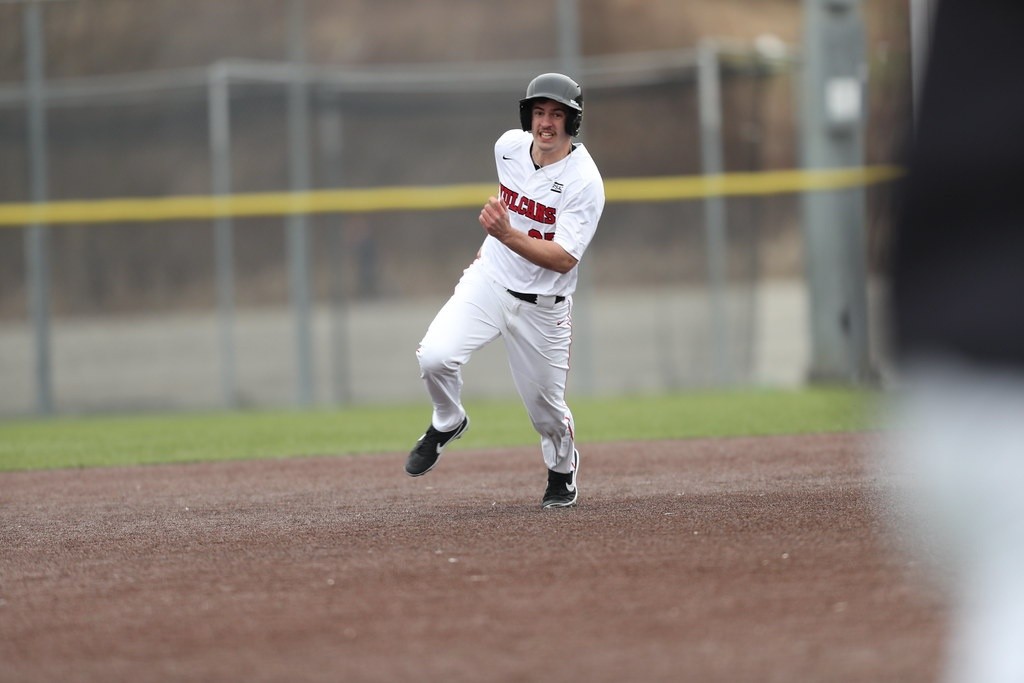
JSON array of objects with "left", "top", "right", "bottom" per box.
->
[
  {"left": 403, "top": 415, "right": 470, "bottom": 477},
  {"left": 542, "top": 448, "right": 580, "bottom": 508}
]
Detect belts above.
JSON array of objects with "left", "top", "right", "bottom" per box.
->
[{"left": 507, "top": 289, "right": 565, "bottom": 305}]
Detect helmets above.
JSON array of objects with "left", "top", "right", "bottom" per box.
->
[{"left": 519, "top": 73, "right": 585, "bottom": 113}]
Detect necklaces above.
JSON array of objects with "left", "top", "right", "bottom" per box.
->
[{"left": 533, "top": 143, "right": 572, "bottom": 181}]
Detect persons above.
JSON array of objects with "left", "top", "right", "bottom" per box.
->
[{"left": 404, "top": 71, "right": 605, "bottom": 509}]
